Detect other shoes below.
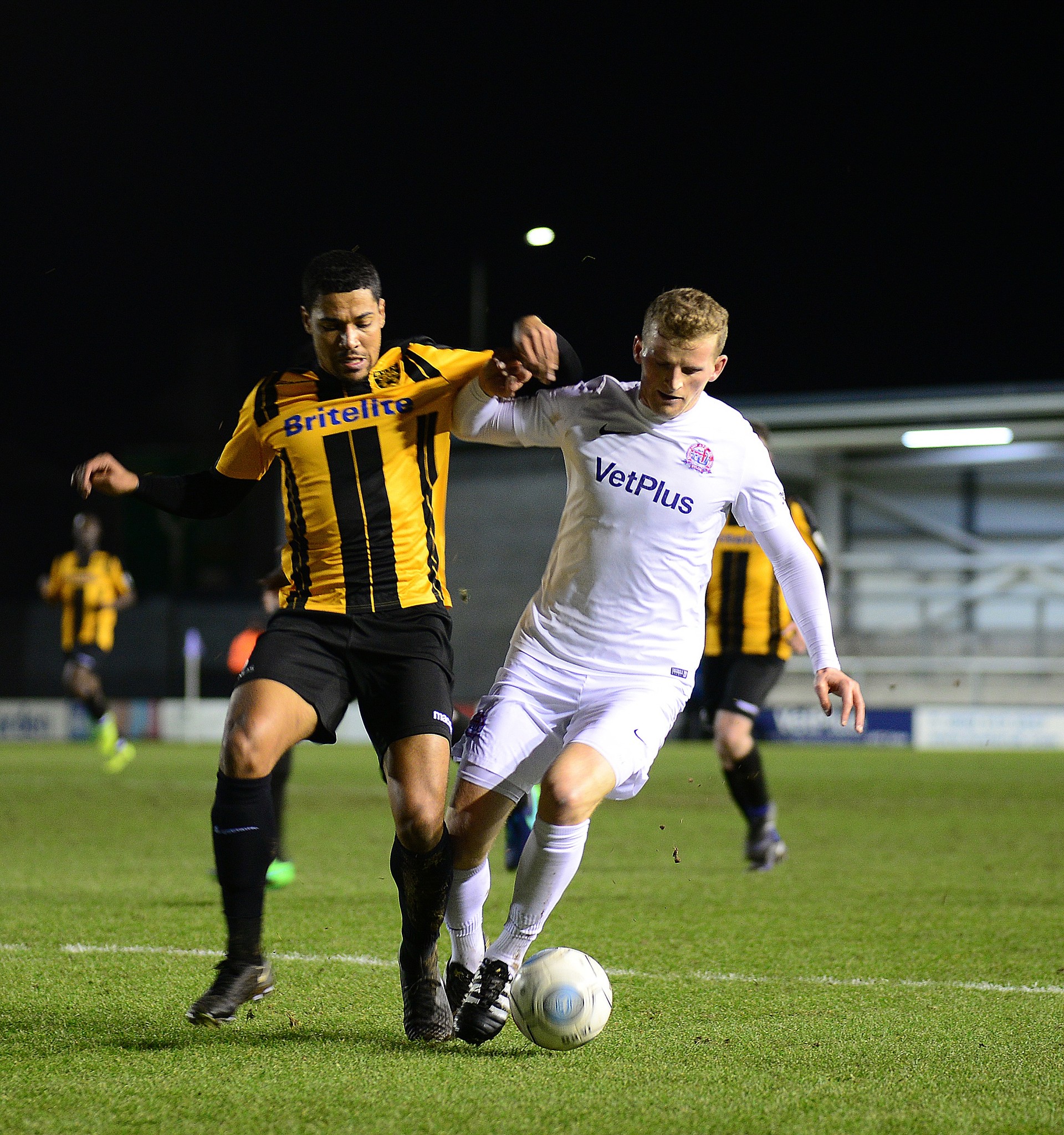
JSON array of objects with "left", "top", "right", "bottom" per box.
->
[
  {"left": 108, "top": 742, "right": 138, "bottom": 776},
  {"left": 100, "top": 714, "right": 118, "bottom": 753},
  {"left": 266, "top": 858, "right": 295, "bottom": 888}
]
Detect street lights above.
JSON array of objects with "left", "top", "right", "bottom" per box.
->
[{"left": 467, "top": 222, "right": 559, "bottom": 350}]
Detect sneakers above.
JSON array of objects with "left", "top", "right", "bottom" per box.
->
[
  {"left": 443, "top": 934, "right": 490, "bottom": 1013},
  {"left": 504, "top": 783, "right": 542, "bottom": 872},
  {"left": 187, "top": 955, "right": 273, "bottom": 1025},
  {"left": 453, "top": 959, "right": 516, "bottom": 1045},
  {"left": 398, "top": 943, "right": 453, "bottom": 1047},
  {"left": 747, "top": 827, "right": 786, "bottom": 866}
]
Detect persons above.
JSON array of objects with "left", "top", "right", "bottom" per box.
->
[
  {"left": 440, "top": 287, "right": 866, "bottom": 1045},
  {"left": 186, "top": 251, "right": 585, "bottom": 1040},
  {"left": 41, "top": 513, "right": 544, "bottom": 893}
]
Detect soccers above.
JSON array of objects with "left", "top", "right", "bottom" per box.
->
[{"left": 507, "top": 951, "right": 611, "bottom": 1047}]
